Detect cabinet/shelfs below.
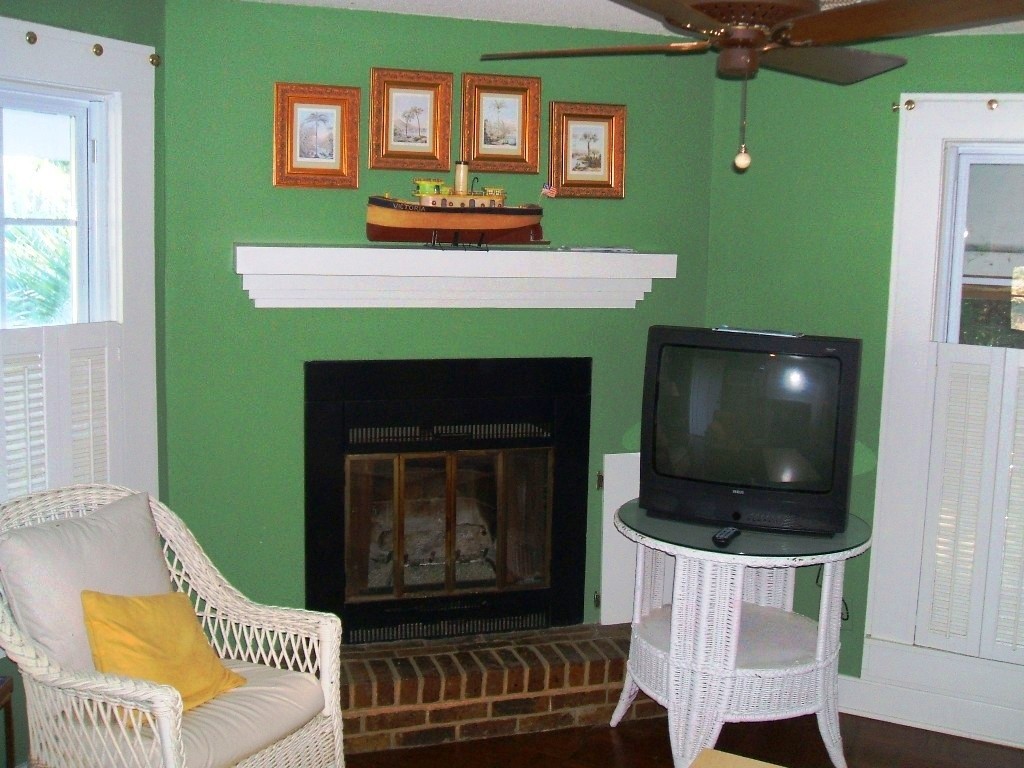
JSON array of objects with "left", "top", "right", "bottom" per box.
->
[{"left": 609, "top": 498, "right": 871, "bottom": 768}]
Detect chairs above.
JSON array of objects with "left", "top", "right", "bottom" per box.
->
[{"left": 0, "top": 482, "right": 346, "bottom": 768}]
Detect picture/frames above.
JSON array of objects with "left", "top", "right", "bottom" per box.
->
[
  {"left": 460, "top": 70, "right": 541, "bottom": 173},
  {"left": 368, "top": 65, "right": 454, "bottom": 171},
  {"left": 548, "top": 100, "right": 627, "bottom": 198},
  {"left": 274, "top": 82, "right": 361, "bottom": 191}
]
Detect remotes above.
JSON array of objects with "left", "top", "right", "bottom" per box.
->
[{"left": 710, "top": 527, "right": 739, "bottom": 547}]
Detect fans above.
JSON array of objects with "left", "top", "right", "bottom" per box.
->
[{"left": 480, "top": 0, "right": 1024, "bottom": 169}]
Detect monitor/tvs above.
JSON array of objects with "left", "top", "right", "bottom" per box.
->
[{"left": 639, "top": 325, "right": 862, "bottom": 539}]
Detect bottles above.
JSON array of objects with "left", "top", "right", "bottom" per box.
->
[{"left": 455, "top": 161, "right": 468, "bottom": 195}]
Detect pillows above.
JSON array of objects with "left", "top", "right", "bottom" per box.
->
[{"left": 81, "top": 590, "right": 247, "bottom": 727}]
